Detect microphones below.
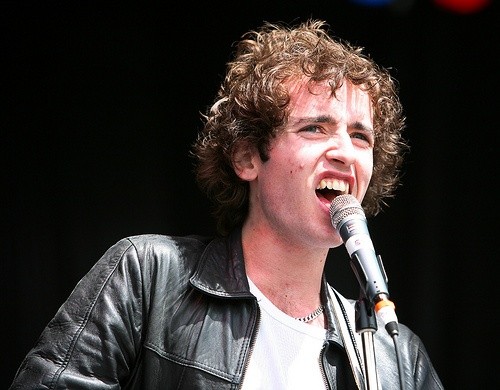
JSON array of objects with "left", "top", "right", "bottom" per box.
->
[{"left": 330, "top": 194, "right": 399, "bottom": 336}]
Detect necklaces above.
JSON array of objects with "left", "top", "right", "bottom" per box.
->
[{"left": 294, "top": 304, "right": 324, "bottom": 323}]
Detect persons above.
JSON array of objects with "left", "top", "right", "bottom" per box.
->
[{"left": 10, "top": 18, "right": 446, "bottom": 389}]
